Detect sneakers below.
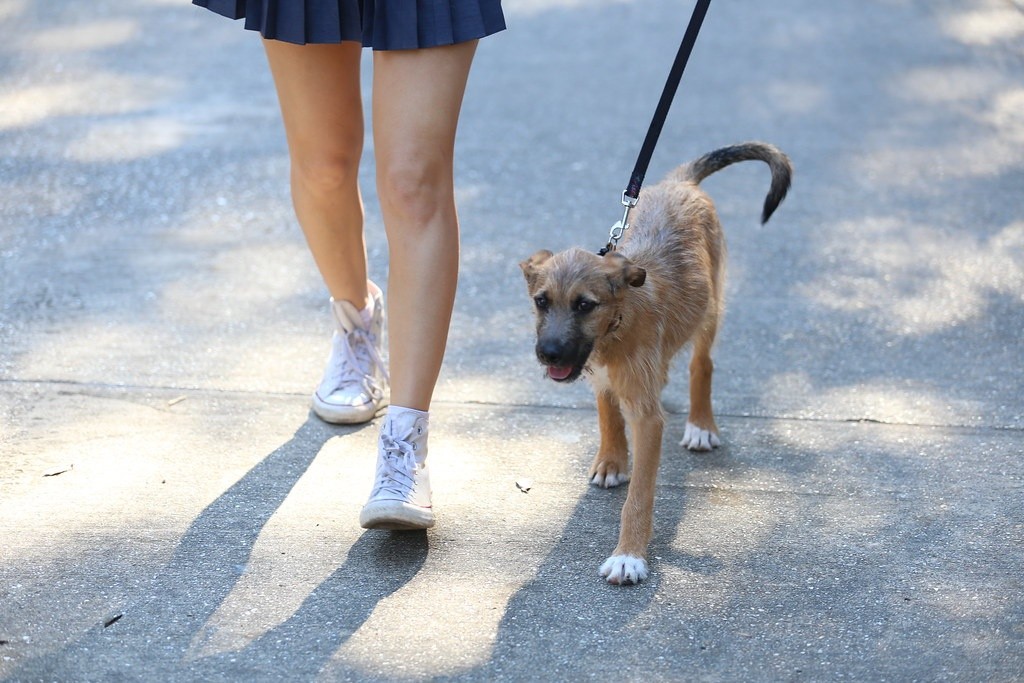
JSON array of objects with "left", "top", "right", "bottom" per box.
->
[
  {"left": 359, "top": 415, "right": 435, "bottom": 528},
  {"left": 312, "top": 278, "right": 391, "bottom": 424}
]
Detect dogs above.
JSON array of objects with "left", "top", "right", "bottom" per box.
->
[{"left": 517, "top": 142, "right": 796, "bottom": 586}]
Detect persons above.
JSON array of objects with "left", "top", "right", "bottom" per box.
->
[{"left": 192, "top": 0, "right": 507, "bottom": 530}]
